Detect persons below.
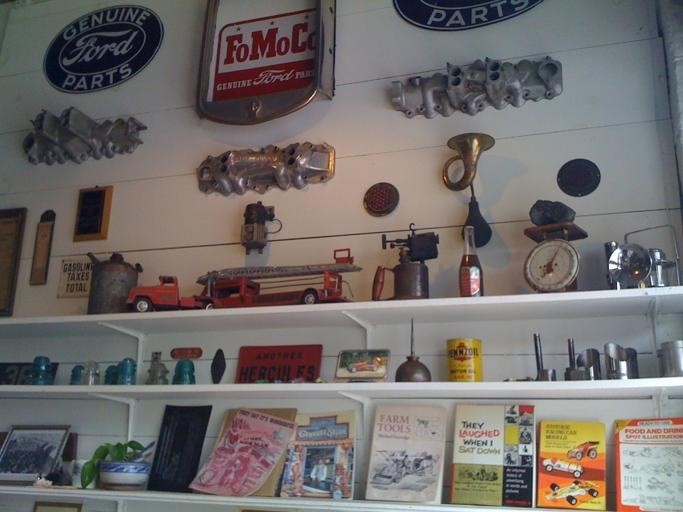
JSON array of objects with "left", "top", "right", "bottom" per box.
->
[{"left": 308, "top": 457, "right": 327, "bottom": 488}]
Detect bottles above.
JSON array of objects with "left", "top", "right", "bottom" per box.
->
[
  {"left": 458, "top": 225, "right": 484, "bottom": 297},
  {"left": 32, "top": 356, "right": 196, "bottom": 385}
]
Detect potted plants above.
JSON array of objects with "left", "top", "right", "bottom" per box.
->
[{"left": 81, "top": 440, "right": 153, "bottom": 490}]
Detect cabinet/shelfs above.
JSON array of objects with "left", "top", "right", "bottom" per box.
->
[{"left": 0, "top": 285, "right": 683, "bottom": 512}]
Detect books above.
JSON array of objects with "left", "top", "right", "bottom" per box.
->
[
  {"left": 364, "top": 403, "right": 447, "bottom": 506},
  {"left": 449, "top": 402, "right": 536, "bottom": 509},
  {"left": 201, "top": 406, "right": 296, "bottom": 496},
  {"left": 278, "top": 408, "right": 355, "bottom": 499},
  {"left": 614, "top": 418, "right": 682, "bottom": 511},
  {"left": 536, "top": 420, "right": 608, "bottom": 511}
]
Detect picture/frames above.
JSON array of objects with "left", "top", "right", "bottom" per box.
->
[
  {"left": 0, "top": 425, "right": 72, "bottom": 484},
  {"left": 336, "top": 349, "right": 391, "bottom": 383}
]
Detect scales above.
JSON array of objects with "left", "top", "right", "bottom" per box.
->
[{"left": 524, "top": 221, "right": 588, "bottom": 293}]
